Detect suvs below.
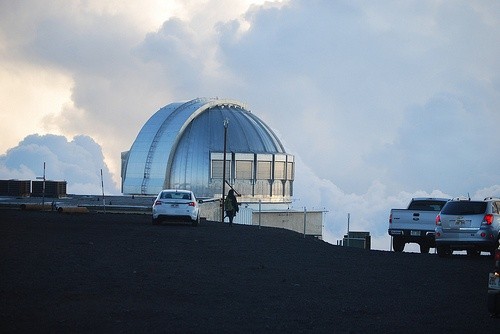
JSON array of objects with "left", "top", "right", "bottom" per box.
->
[{"left": 435, "top": 196, "right": 500, "bottom": 258}]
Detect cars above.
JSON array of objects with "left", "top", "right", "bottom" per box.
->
[
  {"left": 487, "top": 240, "right": 500, "bottom": 319},
  {"left": 151, "top": 188, "right": 204, "bottom": 226}
]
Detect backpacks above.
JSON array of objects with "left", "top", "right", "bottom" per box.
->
[{"left": 225, "top": 198, "right": 234, "bottom": 212}]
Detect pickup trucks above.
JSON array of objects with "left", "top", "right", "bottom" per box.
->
[{"left": 387, "top": 196, "right": 452, "bottom": 254}]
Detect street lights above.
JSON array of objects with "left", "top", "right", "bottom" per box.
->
[{"left": 222, "top": 118, "right": 230, "bottom": 222}]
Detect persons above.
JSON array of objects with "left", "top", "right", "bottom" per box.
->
[{"left": 226, "top": 190, "right": 239, "bottom": 223}]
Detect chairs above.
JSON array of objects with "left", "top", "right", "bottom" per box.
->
[
  {"left": 165, "top": 193, "right": 172, "bottom": 198},
  {"left": 183, "top": 195, "right": 189, "bottom": 199}
]
